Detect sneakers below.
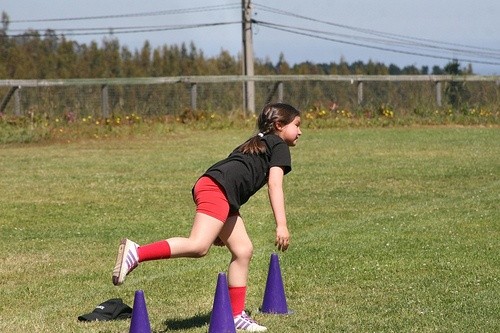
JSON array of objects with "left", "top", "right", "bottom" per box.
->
[
  {"left": 232, "top": 312, "right": 267, "bottom": 333},
  {"left": 113, "top": 237, "right": 137, "bottom": 284}
]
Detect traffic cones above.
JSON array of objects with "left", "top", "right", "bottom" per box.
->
[
  {"left": 129, "top": 289, "right": 152, "bottom": 333},
  {"left": 208, "top": 270, "right": 235, "bottom": 332},
  {"left": 258, "top": 254, "right": 291, "bottom": 314}
]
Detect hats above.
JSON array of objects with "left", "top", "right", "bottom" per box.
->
[{"left": 79, "top": 298, "right": 131, "bottom": 322}]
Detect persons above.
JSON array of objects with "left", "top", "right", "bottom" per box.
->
[{"left": 111, "top": 101, "right": 303, "bottom": 333}]
